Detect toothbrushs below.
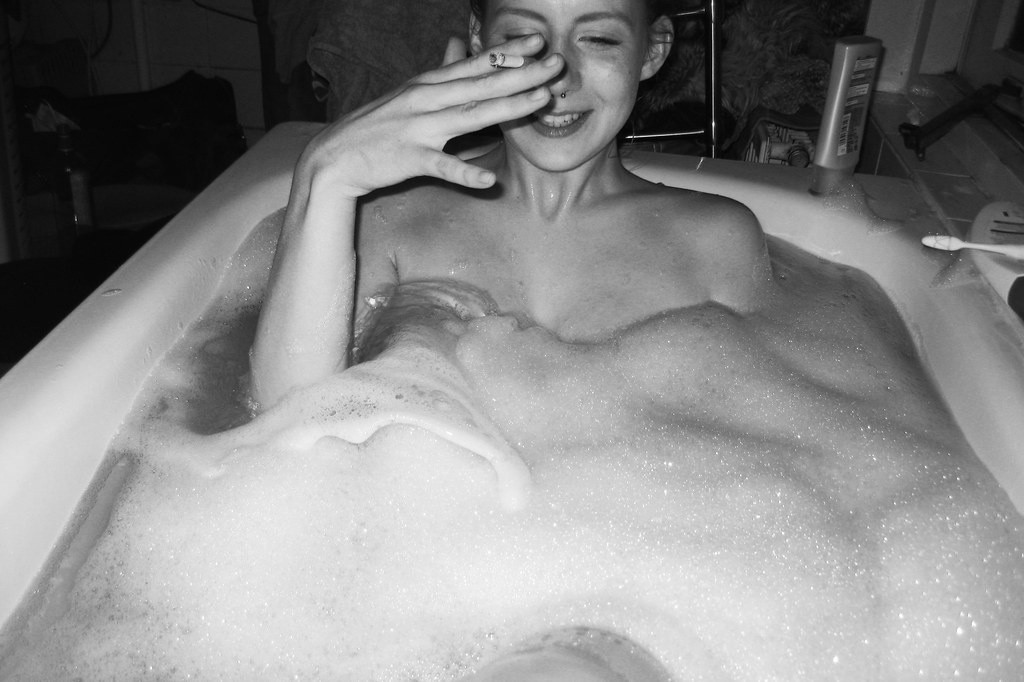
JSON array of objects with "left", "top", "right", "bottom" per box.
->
[{"left": 921, "top": 235, "right": 1024, "bottom": 262}]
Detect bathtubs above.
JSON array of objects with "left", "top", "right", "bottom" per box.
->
[{"left": 0, "top": 119, "right": 1024, "bottom": 681}]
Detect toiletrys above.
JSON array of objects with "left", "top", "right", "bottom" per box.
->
[{"left": 808, "top": 36, "right": 883, "bottom": 196}]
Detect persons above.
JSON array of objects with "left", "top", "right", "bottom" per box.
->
[{"left": 252, "top": 0, "right": 772, "bottom": 425}]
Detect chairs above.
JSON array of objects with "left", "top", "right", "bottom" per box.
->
[{"left": 59, "top": 73, "right": 246, "bottom": 273}]
